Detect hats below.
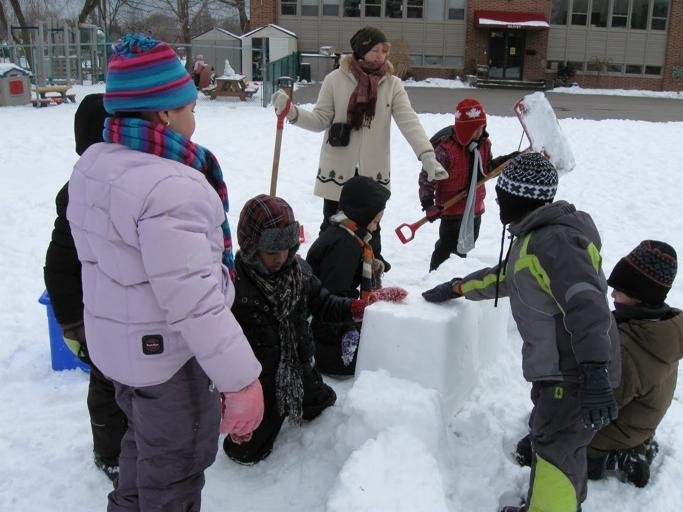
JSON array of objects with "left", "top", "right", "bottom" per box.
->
[
  {"left": 491, "top": 151, "right": 559, "bottom": 308},
  {"left": 348, "top": 26, "right": 390, "bottom": 61},
  {"left": 453, "top": 97, "right": 489, "bottom": 147},
  {"left": 605, "top": 239, "right": 679, "bottom": 308},
  {"left": 237, "top": 194, "right": 305, "bottom": 279},
  {"left": 102, "top": 33, "right": 199, "bottom": 117}
]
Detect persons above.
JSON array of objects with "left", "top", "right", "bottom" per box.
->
[
  {"left": 422, "top": 153, "right": 622, "bottom": 512},
  {"left": 66, "top": 35, "right": 264, "bottom": 512},
  {"left": 43, "top": 92, "right": 128, "bottom": 484},
  {"left": 306, "top": 177, "right": 392, "bottom": 376},
  {"left": 225, "top": 194, "right": 408, "bottom": 467},
  {"left": 271, "top": 27, "right": 449, "bottom": 290},
  {"left": 419, "top": 99, "right": 520, "bottom": 273},
  {"left": 513, "top": 240, "right": 683, "bottom": 488}
]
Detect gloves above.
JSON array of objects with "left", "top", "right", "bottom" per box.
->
[
  {"left": 417, "top": 151, "right": 450, "bottom": 183},
  {"left": 350, "top": 285, "right": 407, "bottom": 325},
  {"left": 490, "top": 151, "right": 520, "bottom": 174},
  {"left": 270, "top": 89, "right": 299, "bottom": 122},
  {"left": 216, "top": 376, "right": 265, "bottom": 446},
  {"left": 425, "top": 203, "right": 447, "bottom": 222},
  {"left": 578, "top": 367, "right": 619, "bottom": 434},
  {"left": 421, "top": 277, "right": 464, "bottom": 306},
  {"left": 340, "top": 330, "right": 359, "bottom": 369},
  {"left": 57, "top": 321, "right": 93, "bottom": 368}
]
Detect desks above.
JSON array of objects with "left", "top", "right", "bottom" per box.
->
[
  {"left": 216, "top": 74, "right": 246, "bottom": 91},
  {"left": 37, "top": 85, "right": 69, "bottom": 107}
]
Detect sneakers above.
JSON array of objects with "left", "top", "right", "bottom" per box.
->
[{"left": 616, "top": 433, "right": 659, "bottom": 490}]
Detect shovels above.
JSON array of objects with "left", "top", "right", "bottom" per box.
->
[{"left": 395, "top": 90, "right": 575, "bottom": 244}]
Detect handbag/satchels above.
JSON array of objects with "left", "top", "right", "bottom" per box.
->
[{"left": 325, "top": 122, "right": 353, "bottom": 147}]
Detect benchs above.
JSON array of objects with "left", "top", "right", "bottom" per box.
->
[
  {"left": 31, "top": 99, "right": 51, "bottom": 107},
  {"left": 46, "top": 94, "right": 76, "bottom": 105},
  {"left": 245, "top": 86, "right": 259, "bottom": 97},
  {"left": 202, "top": 85, "right": 216, "bottom": 100}
]
